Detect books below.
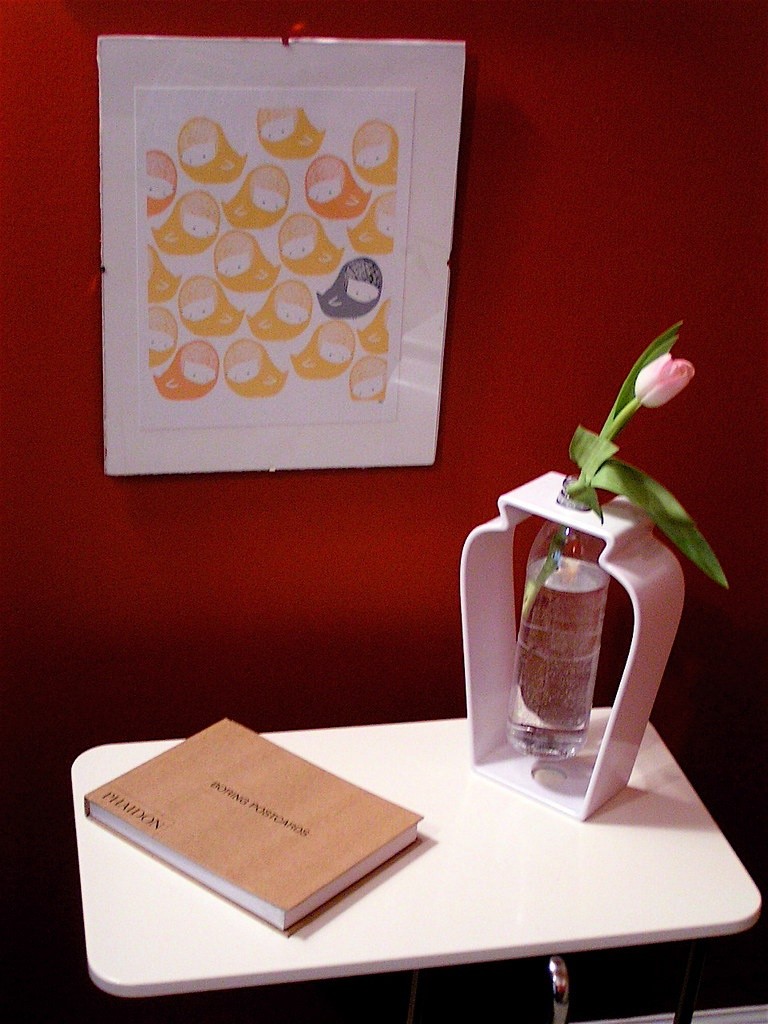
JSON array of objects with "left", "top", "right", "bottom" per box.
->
[{"left": 83, "top": 718, "right": 425, "bottom": 938}]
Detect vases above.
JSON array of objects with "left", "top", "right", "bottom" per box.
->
[{"left": 504, "top": 522, "right": 608, "bottom": 760}]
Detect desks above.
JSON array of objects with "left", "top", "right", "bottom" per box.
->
[{"left": 72, "top": 706, "right": 762, "bottom": 1024}]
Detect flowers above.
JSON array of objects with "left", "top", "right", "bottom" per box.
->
[{"left": 562, "top": 320, "right": 731, "bottom": 592}]
